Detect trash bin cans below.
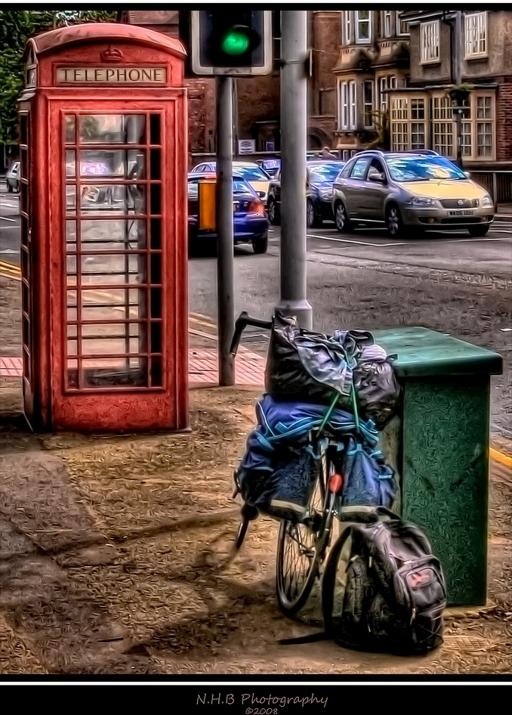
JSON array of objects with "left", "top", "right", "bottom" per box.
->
[{"left": 354, "top": 327, "right": 503, "bottom": 606}]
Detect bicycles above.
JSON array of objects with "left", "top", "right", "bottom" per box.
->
[{"left": 226, "top": 308, "right": 374, "bottom": 613}]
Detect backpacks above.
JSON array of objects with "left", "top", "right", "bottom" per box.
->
[{"left": 278, "top": 507, "right": 447, "bottom": 656}]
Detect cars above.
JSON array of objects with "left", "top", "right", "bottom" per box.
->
[
  {"left": 257, "top": 157, "right": 280, "bottom": 173},
  {"left": 5, "top": 160, "right": 20, "bottom": 191},
  {"left": 329, "top": 145, "right": 497, "bottom": 237},
  {"left": 265, "top": 159, "right": 349, "bottom": 228},
  {"left": 189, "top": 160, "right": 273, "bottom": 206},
  {"left": 187, "top": 170, "right": 270, "bottom": 255},
  {"left": 55, "top": 161, "right": 138, "bottom": 204}
]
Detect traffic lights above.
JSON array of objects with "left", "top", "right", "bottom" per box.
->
[{"left": 190, "top": 10, "right": 273, "bottom": 74}]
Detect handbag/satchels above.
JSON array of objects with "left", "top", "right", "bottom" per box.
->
[{"left": 234, "top": 393, "right": 396, "bottom": 521}]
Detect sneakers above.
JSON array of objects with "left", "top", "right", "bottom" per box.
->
[
  {"left": 334, "top": 329, "right": 386, "bottom": 363},
  {"left": 270, "top": 455, "right": 311, "bottom": 513}
]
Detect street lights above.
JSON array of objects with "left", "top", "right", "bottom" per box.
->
[{"left": 444, "top": 88, "right": 470, "bottom": 169}]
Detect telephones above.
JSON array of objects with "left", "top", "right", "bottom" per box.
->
[{"left": 128, "top": 154, "right": 148, "bottom": 220}]
